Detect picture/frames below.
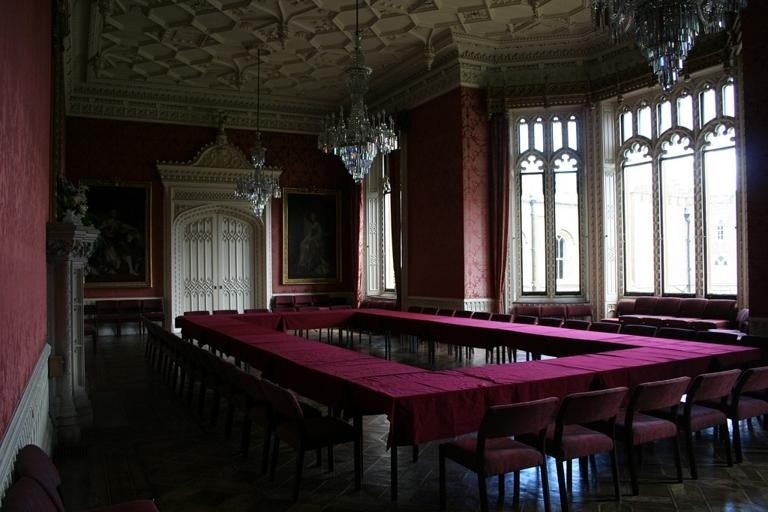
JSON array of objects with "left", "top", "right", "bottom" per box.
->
[
  {"left": 280, "top": 186, "right": 344, "bottom": 285},
  {"left": 83, "top": 176, "right": 153, "bottom": 290}
]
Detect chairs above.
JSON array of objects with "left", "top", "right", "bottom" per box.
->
[{"left": 1, "top": 445, "right": 158, "bottom": 510}]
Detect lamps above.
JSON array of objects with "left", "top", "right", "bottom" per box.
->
[
  {"left": 234, "top": 2, "right": 399, "bottom": 220},
  {"left": 590, "top": 0, "right": 746, "bottom": 91}
]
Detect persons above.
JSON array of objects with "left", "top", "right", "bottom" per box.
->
[
  {"left": 96, "top": 209, "right": 143, "bottom": 277},
  {"left": 296, "top": 209, "right": 335, "bottom": 276}
]
